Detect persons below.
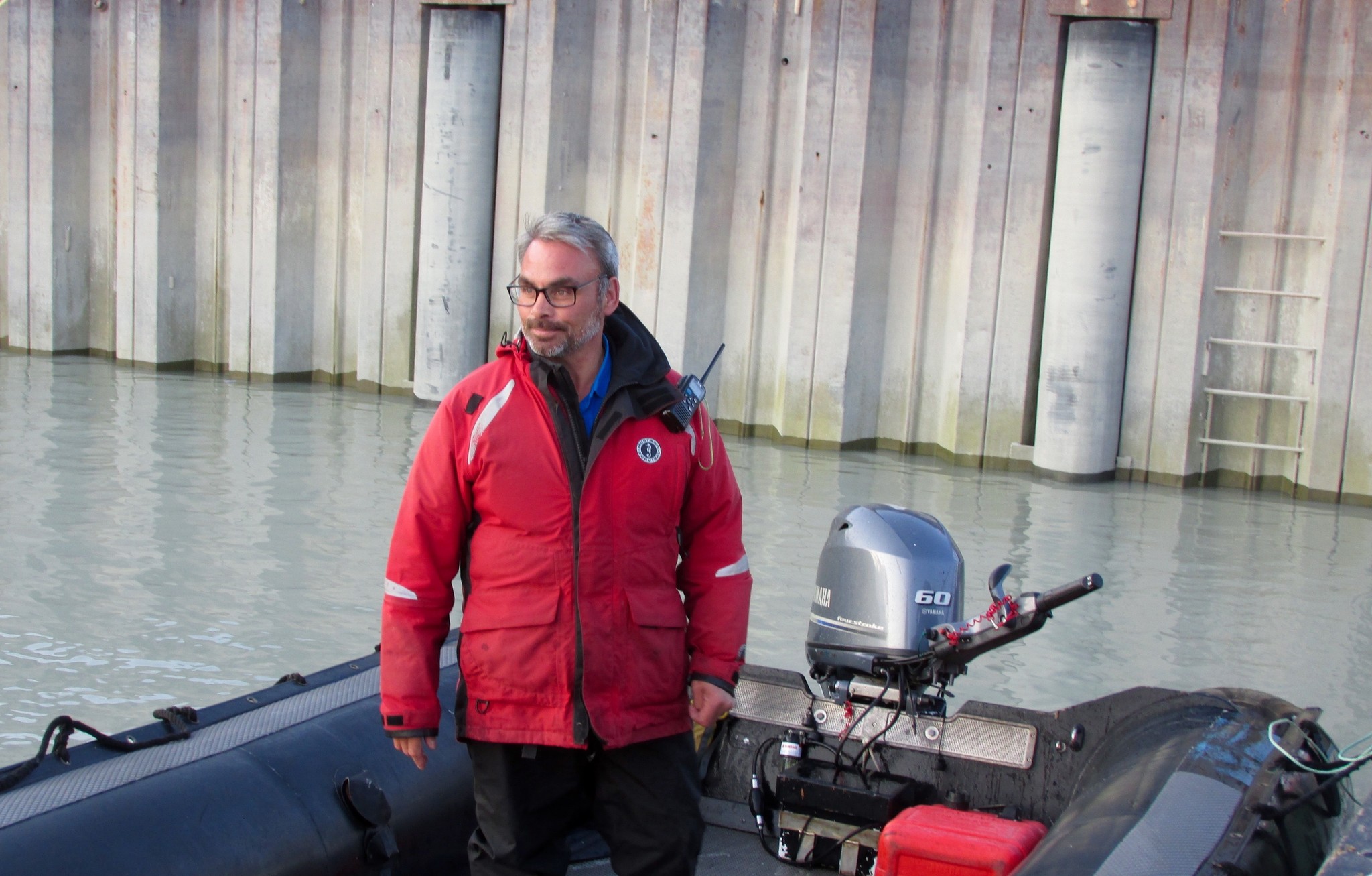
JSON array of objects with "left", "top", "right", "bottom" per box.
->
[{"left": 379, "top": 211, "right": 752, "bottom": 875}]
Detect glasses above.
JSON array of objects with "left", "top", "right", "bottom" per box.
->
[{"left": 508, "top": 276, "right": 603, "bottom": 308}]
